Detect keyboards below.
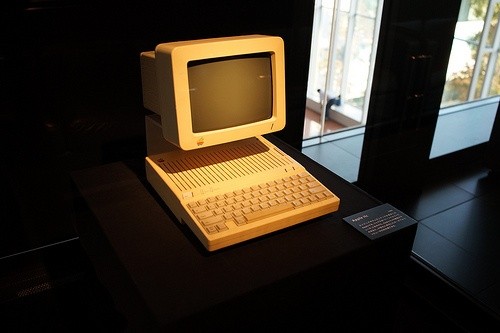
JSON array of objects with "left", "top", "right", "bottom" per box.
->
[{"left": 181, "top": 170, "right": 341, "bottom": 251}]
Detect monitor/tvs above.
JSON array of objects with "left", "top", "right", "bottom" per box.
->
[{"left": 140, "top": 34, "right": 286, "bottom": 151}]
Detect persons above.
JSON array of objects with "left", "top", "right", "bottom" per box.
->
[{"left": 318, "top": 89, "right": 341, "bottom": 122}]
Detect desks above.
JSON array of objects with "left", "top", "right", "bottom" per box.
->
[{"left": 65, "top": 136, "right": 417, "bottom": 333}]
